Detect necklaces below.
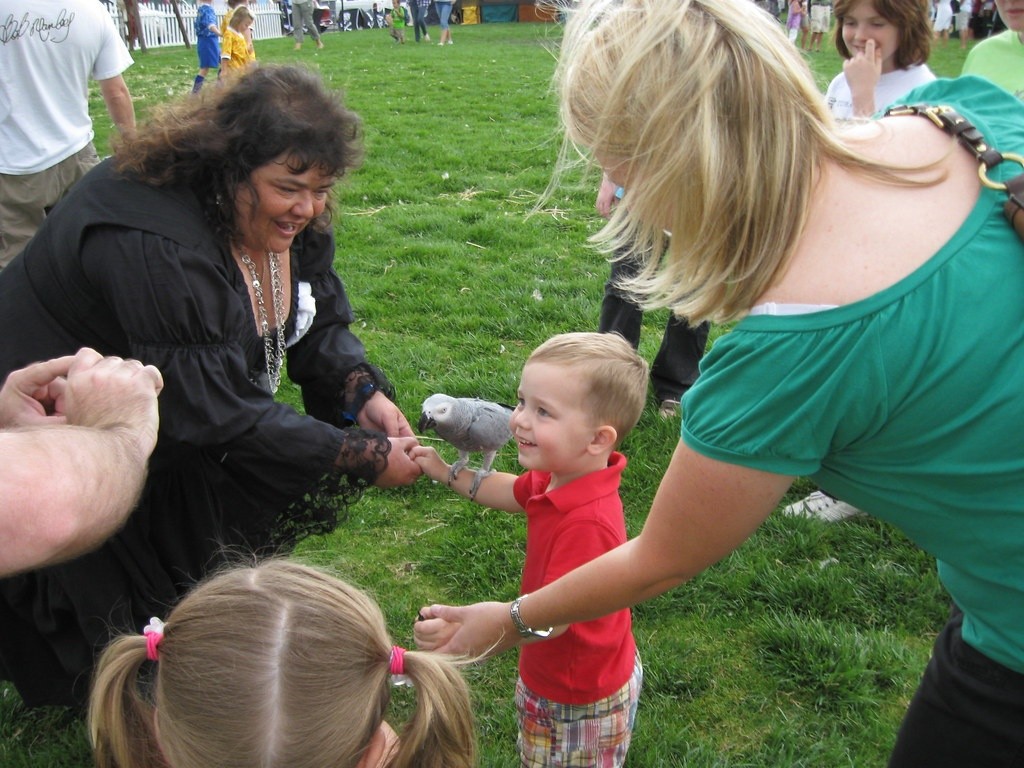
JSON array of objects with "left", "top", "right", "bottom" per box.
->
[{"left": 241, "top": 252, "right": 287, "bottom": 393}]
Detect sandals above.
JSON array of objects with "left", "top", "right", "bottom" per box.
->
[{"left": 437, "top": 42, "right": 445, "bottom": 46}]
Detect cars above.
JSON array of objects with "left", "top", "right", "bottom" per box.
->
[{"left": 281, "top": 0, "right": 334, "bottom": 33}]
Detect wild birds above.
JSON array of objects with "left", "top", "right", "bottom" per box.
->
[{"left": 419, "top": 394, "right": 518, "bottom": 500}]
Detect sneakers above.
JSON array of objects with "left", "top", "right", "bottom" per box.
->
[{"left": 781, "top": 488, "right": 865, "bottom": 523}]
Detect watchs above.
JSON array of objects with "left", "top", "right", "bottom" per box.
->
[{"left": 510, "top": 594, "right": 554, "bottom": 639}]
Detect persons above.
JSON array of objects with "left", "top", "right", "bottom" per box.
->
[
  {"left": 193, "top": 0, "right": 223, "bottom": 93},
  {"left": 407, "top": 0, "right": 431, "bottom": 42},
  {"left": 221, "top": 6, "right": 254, "bottom": 90},
  {"left": 0, "top": 0, "right": 135, "bottom": 273},
  {"left": 430, "top": 0, "right": 1024, "bottom": 768},
  {"left": 220, "top": 0, "right": 255, "bottom": 76},
  {"left": 786, "top": 0, "right": 831, "bottom": 52},
  {"left": 292, "top": 0, "right": 323, "bottom": 49},
  {"left": 0, "top": 65, "right": 424, "bottom": 709},
  {"left": 390, "top": 0, "right": 405, "bottom": 44},
  {"left": 88, "top": 559, "right": 477, "bottom": 768},
  {"left": 595, "top": 171, "right": 711, "bottom": 422},
  {"left": 781, "top": 0, "right": 937, "bottom": 524},
  {"left": 408, "top": 332, "right": 650, "bottom": 768},
  {"left": 929, "top": 0, "right": 1008, "bottom": 49},
  {"left": 0, "top": 347, "right": 164, "bottom": 576},
  {"left": 961, "top": 0, "right": 1024, "bottom": 102},
  {"left": 433, "top": 0, "right": 456, "bottom": 46}
]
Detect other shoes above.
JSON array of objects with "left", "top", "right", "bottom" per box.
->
[
  {"left": 294, "top": 43, "right": 301, "bottom": 51},
  {"left": 401, "top": 37, "right": 405, "bottom": 43},
  {"left": 447, "top": 40, "right": 453, "bottom": 45},
  {"left": 317, "top": 42, "right": 324, "bottom": 49},
  {"left": 658, "top": 399, "right": 681, "bottom": 420},
  {"left": 425, "top": 33, "right": 430, "bottom": 42},
  {"left": 395, "top": 38, "right": 400, "bottom": 44}
]
